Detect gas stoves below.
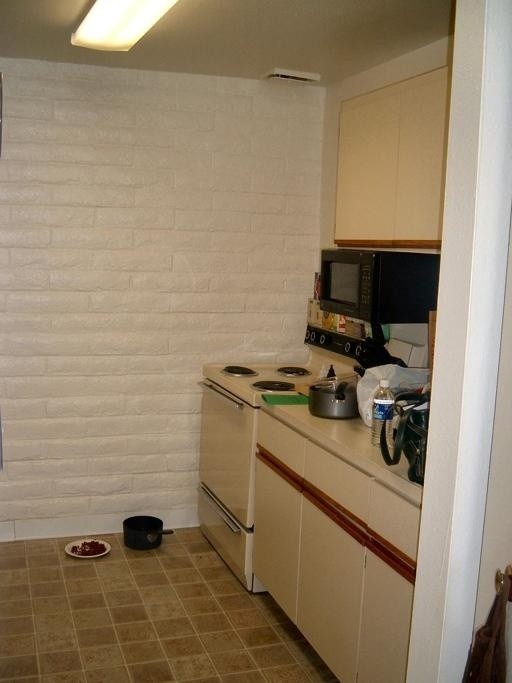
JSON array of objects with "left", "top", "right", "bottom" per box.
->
[{"left": 202, "top": 362, "right": 324, "bottom": 408}]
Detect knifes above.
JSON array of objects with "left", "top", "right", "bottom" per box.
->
[{"left": 355, "top": 336, "right": 391, "bottom": 369}]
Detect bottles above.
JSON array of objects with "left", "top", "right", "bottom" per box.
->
[{"left": 371, "top": 379, "right": 394, "bottom": 448}]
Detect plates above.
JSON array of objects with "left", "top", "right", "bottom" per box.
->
[{"left": 65, "top": 538, "right": 112, "bottom": 560}]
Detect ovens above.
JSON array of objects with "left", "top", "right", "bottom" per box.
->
[{"left": 197, "top": 377, "right": 268, "bottom": 594}]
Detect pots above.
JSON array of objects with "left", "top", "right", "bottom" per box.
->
[
  {"left": 123, "top": 516, "right": 174, "bottom": 551},
  {"left": 309, "top": 381, "right": 358, "bottom": 420}
]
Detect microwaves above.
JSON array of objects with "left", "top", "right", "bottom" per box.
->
[{"left": 319, "top": 248, "right": 440, "bottom": 324}]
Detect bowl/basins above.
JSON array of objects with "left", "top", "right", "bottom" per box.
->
[{"left": 316, "top": 371, "right": 360, "bottom": 389}]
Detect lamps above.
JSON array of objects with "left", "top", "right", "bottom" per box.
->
[{"left": 70, "top": 0, "right": 179, "bottom": 52}]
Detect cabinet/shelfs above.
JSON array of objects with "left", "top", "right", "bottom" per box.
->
[
  {"left": 252, "top": 395, "right": 374, "bottom": 683},
  {"left": 356, "top": 453, "right": 423, "bottom": 683},
  {"left": 333, "top": 64, "right": 449, "bottom": 247}
]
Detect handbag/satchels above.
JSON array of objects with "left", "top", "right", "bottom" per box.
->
[
  {"left": 462, "top": 574, "right": 511, "bottom": 683},
  {"left": 379, "top": 391, "right": 432, "bottom": 486}
]
